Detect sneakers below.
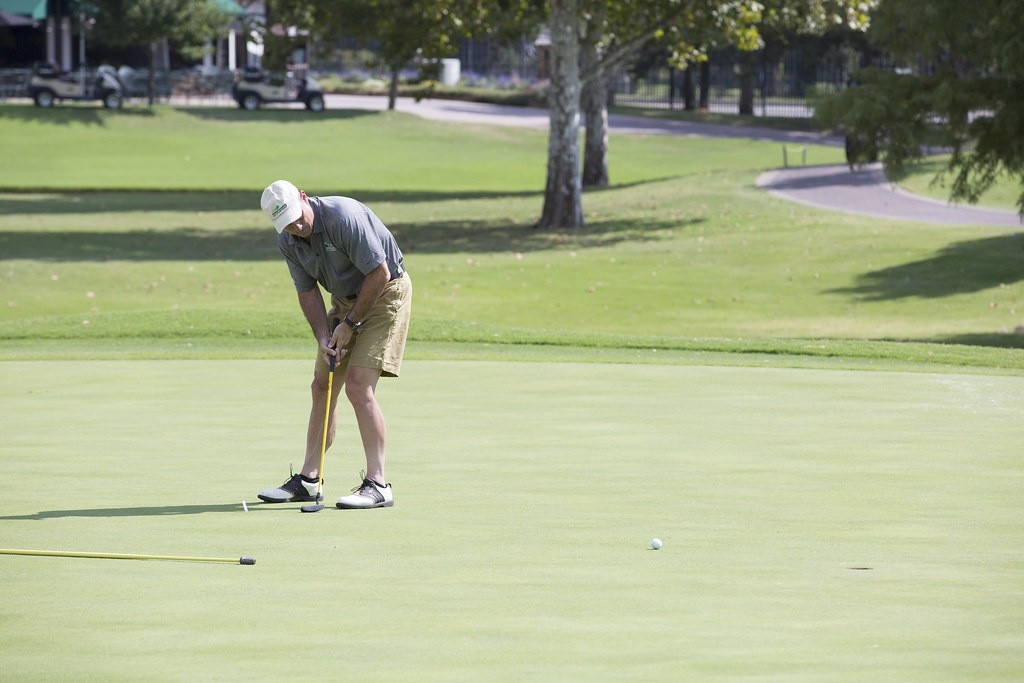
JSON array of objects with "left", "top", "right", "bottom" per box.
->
[
  {"left": 257, "top": 463, "right": 324, "bottom": 503},
  {"left": 336, "top": 469, "right": 394, "bottom": 509}
]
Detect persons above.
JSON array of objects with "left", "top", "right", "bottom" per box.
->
[{"left": 257, "top": 180, "right": 413, "bottom": 508}]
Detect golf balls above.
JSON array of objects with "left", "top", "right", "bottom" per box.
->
[{"left": 651, "top": 538, "right": 662, "bottom": 549}]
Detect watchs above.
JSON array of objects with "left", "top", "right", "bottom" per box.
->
[{"left": 344, "top": 315, "right": 361, "bottom": 330}]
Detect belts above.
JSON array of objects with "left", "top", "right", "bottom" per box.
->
[{"left": 345, "top": 261, "right": 406, "bottom": 301}]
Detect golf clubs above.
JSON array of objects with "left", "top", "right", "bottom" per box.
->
[
  {"left": 1, "top": 548, "right": 257, "bottom": 565},
  {"left": 301, "top": 317, "right": 341, "bottom": 513}
]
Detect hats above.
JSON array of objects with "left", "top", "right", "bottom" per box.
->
[{"left": 261, "top": 179, "right": 302, "bottom": 234}]
